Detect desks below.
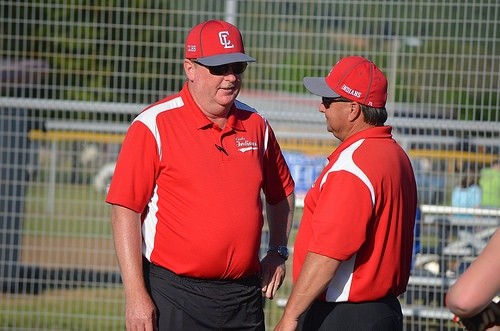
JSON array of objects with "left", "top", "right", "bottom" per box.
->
[{"left": 419, "top": 203, "right": 500, "bottom": 254}]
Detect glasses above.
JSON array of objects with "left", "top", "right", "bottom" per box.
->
[
  {"left": 193, "top": 60, "right": 248, "bottom": 76},
  {"left": 322, "top": 97, "right": 351, "bottom": 109}
]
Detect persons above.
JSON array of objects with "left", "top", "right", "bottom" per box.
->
[
  {"left": 274, "top": 56, "right": 418, "bottom": 331},
  {"left": 105, "top": 19, "right": 295, "bottom": 331},
  {"left": 450, "top": 161, "right": 481, "bottom": 208},
  {"left": 445, "top": 226, "right": 500, "bottom": 331}
]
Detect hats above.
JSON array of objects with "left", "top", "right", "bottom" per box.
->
[
  {"left": 303, "top": 55, "right": 388, "bottom": 108},
  {"left": 185, "top": 19, "right": 256, "bottom": 66}
]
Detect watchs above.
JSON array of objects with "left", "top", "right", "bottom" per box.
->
[{"left": 269, "top": 242, "right": 289, "bottom": 260}]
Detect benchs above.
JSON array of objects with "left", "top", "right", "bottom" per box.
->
[{"left": 275, "top": 274, "right": 461, "bottom": 324}]
